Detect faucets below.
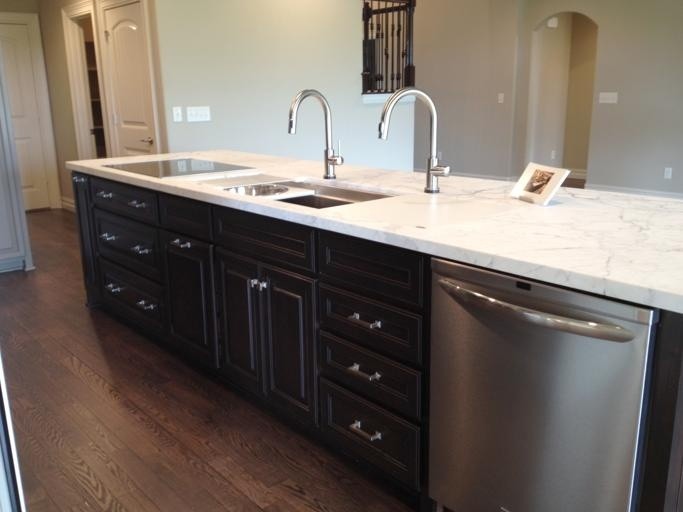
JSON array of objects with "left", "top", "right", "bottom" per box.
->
[
  {"left": 378, "top": 87, "right": 450, "bottom": 193},
  {"left": 288, "top": 89, "right": 343, "bottom": 180}
]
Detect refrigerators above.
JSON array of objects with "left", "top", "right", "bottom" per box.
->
[{"left": 430, "top": 257, "right": 660, "bottom": 512}]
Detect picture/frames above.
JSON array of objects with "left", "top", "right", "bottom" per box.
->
[{"left": 509, "top": 162, "right": 570, "bottom": 206}]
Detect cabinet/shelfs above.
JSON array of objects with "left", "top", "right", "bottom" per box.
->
[
  {"left": 315, "top": 228, "right": 428, "bottom": 510},
  {"left": 70, "top": 170, "right": 171, "bottom": 354},
  {"left": 85, "top": 38, "right": 106, "bottom": 158},
  {"left": 156, "top": 190, "right": 221, "bottom": 382},
  {"left": 212, "top": 205, "right": 319, "bottom": 443}
]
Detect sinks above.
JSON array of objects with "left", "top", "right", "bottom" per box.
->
[
  {"left": 276, "top": 186, "right": 399, "bottom": 210},
  {"left": 187, "top": 172, "right": 315, "bottom": 201}
]
